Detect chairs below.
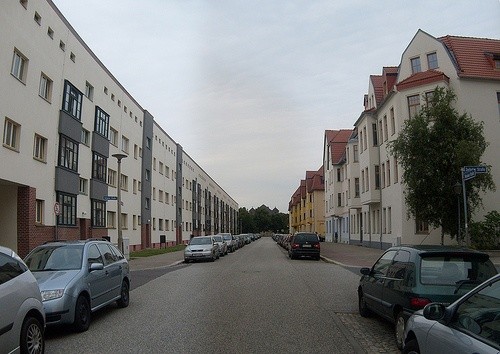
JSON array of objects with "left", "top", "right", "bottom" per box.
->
[{"left": 438, "top": 262, "right": 461, "bottom": 282}]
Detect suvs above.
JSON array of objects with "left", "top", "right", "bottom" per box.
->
[
  {"left": 288, "top": 231, "right": 320, "bottom": 260},
  {"left": 23, "top": 237, "right": 131, "bottom": 333}
]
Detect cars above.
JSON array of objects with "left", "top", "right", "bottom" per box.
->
[
  {"left": 272, "top": 234, "right": 292, "bottom": 250},
  {"left": 0, "top": 246, "right": 45, "bottom": 354},
  {"left": 184, "top": 233, "right": 262, "bottom": 263},
  {"left": 357, "top": 244, "right": 498, "bottom": 352},
  {"left": 403, "top": 273, "right": 500, "bottom": 354}
]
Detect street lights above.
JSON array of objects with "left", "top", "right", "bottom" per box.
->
[{"left": 111, "top": 154, "right": 128, "bottom": 254}]
